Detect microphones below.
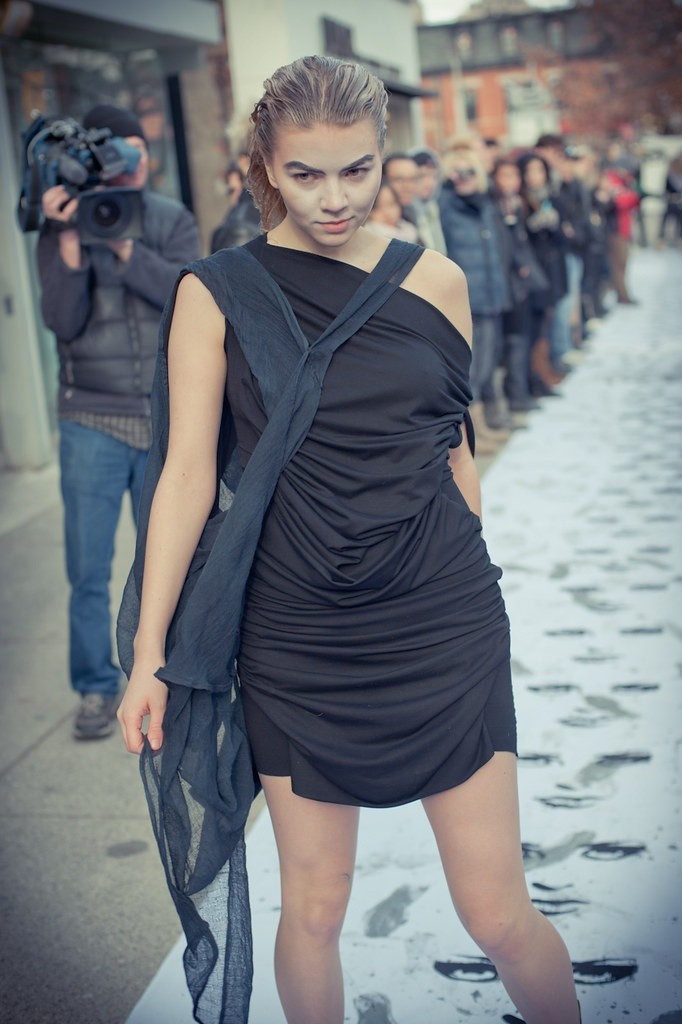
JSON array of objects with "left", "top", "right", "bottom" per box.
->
[{"left": 57, "top": 155, "right": 86, "bottom": 184}]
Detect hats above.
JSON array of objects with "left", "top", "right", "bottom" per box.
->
[{"left": 84, "top": 102, "right": 154, "bottom": 147}]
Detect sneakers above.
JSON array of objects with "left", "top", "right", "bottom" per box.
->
[{"left": 73, "top": 673, "right": 129, "bottom": 745}]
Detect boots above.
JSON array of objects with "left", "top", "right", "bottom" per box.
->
[{"left": 467, "top": 330, "right": 571, "bottom": 459}]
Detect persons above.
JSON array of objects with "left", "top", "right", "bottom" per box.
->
[
  {"left": 117, "top": 56, "right": 579, "bottom": 1024},
  {"left": 211, "top": 121, "right": 681, "bottom": 430},
  {"left": 35, "top": 109, "right": 201, "bottom": 742}
]
